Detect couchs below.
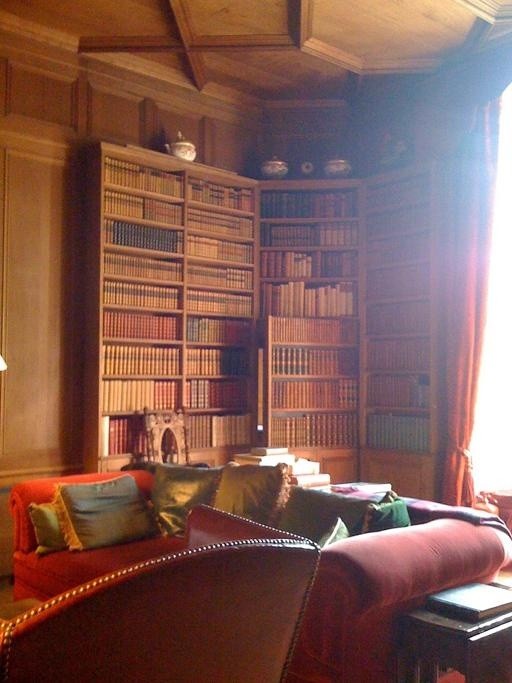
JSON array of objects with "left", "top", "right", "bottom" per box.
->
[{"left": 8, "top": 464, "right": 512, "bottom": 683}]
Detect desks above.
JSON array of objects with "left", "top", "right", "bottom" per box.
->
[{"left": 397, "top": 583, "right": 512, "bottom": 683}]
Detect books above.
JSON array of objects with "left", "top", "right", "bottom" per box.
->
[
  {"left": 234, "top": 447, "right": 392, "bottom": 498},
  {"left": 427, "top": 582, "right": 511, "bottom": 620},
  {"left": 101, "top": 156, "right": 254, "bottom": 456},
  {"left": 260, "top": 178, "right": 433, "bottom": 449}
]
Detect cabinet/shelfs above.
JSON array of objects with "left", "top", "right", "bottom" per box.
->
[
  {"left": 268, "top": 320, "right": 358, "bottom": 452},
  {"left": 260, "top": 183, "right": 361, "bottom": 317},
  {"left": 361, "top": 168, "right": 438, "bottom": 490},
  {"left": 79, "top": 148, "right": 255, "bottom": 449}
]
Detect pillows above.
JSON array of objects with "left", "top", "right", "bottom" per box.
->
[
  {"left": 149, "top": 467, "right": 228, "bottom": 534},
  {"left": 358, "top": 499, "right": 409, "bottom": 535},
  {"left": 30, "top": 502, "right": 65, "bottom": 552},
  {"left": 55, "top": 474, "right": 149, "bottom": 550},
  {"left": 318, "top": 517, "right": 349, "bottom": 549},
  {"left": 213, "top": 464, "right": 291, "bottom": 524}
]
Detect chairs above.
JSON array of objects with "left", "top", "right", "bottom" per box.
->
[{"left": 0, "top": 503, "right": 321, "bottom": 683}]
